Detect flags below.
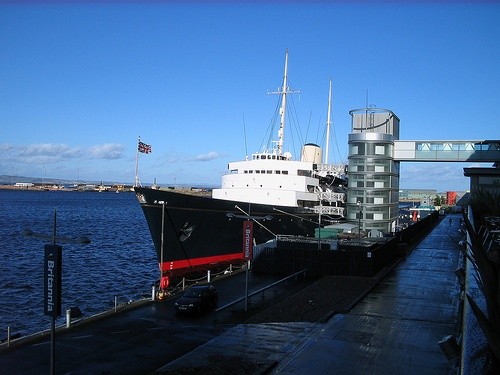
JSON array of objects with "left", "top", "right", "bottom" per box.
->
[{"left": 138, "top": 142, "right": 151, "bottom": 154}]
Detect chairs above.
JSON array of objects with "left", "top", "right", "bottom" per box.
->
[{"left": 477, "top": 217, "right": 500, "bottom": 256}]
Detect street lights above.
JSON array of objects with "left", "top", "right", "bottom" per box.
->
[{"left": 356, "top": 201, "right": 362, "bottom": 241}]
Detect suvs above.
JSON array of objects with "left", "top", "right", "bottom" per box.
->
[{"left": 174, "top": 284, "right": 220, "bottom": 319}]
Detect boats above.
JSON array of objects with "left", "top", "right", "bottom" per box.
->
[{"left": 132, "top": 51, "right": 350, "bottom": 292}]
